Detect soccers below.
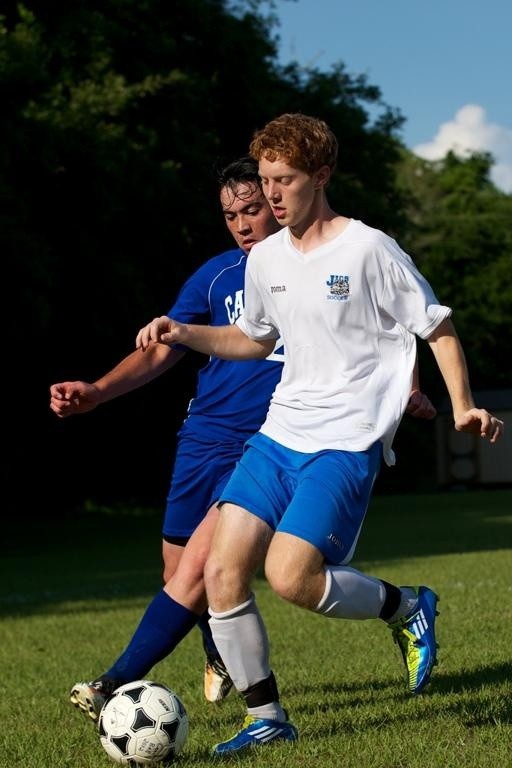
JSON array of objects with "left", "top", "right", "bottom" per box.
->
[{"left": 99, "top": 679, "right": 189, "bottom": 766}]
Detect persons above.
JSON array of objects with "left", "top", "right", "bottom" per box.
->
[
  {"left": 136, "top": 115, "right": 505, "bottom": 755},
  {"left": 50, "top": 158, "right": 436, "bottom": 724}
]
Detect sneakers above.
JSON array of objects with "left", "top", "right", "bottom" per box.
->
[
  {"left": 70, "top": 681, "right": 107, "bottom": 724},
  {"left": 210, "top": 706, "right": 297, "bottom": 754},
  {"left": 203, "top": 655, "right": 233, "bottom": 703},
  {"left": 387, "top": 585, "right": 440, "bottom": 692}
]
